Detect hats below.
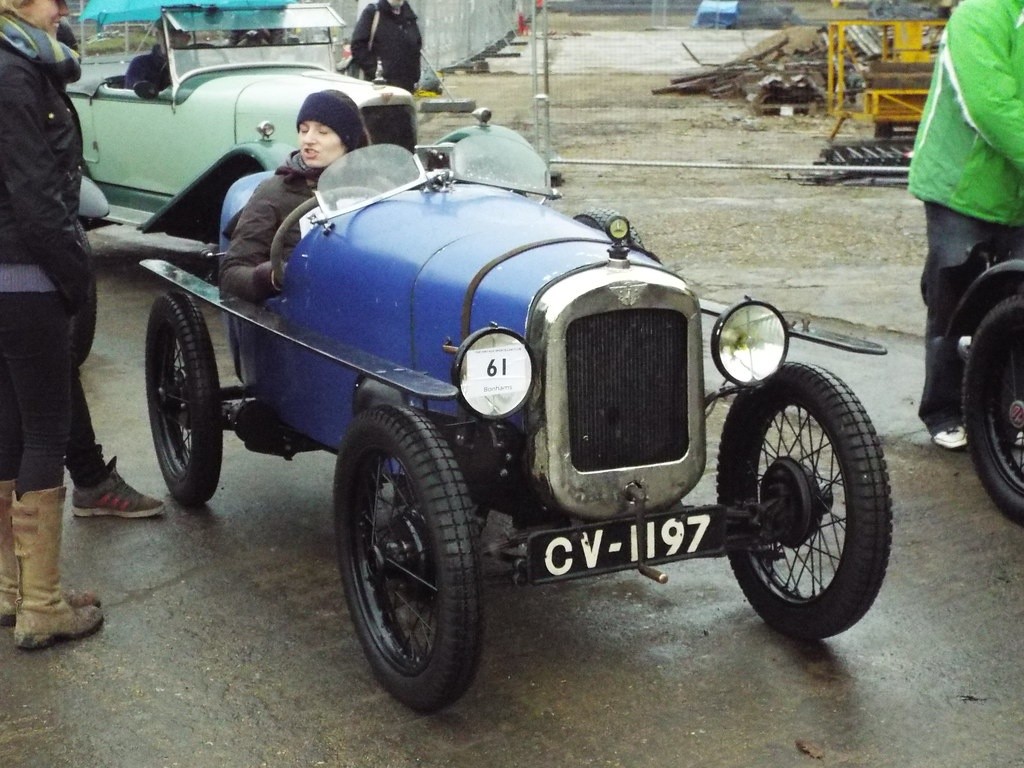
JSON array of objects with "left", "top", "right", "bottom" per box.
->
[
  {"left": 297, "top": 89, "right": 363, "bottom": 152},
  {"left": 155, "top": 16, "right": 189, "bottom": 33}
]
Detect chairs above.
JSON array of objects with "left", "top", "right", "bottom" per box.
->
[{"left": 220, "top": 171, "right": 280, "bottom": 378}]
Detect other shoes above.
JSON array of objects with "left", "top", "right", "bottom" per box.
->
[
  {"left": 1014, "top": 432, "right": 1024, "bottom": 445},
  {"left": 934, "top": 424, "right": 968, "bottom": 450}
]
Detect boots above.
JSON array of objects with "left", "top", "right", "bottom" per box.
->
[
  {"left": 11, "top": 486, "right": 103, "bottom": 648},
  {"left": 0, "top": 478, "right": 101, "bottom": 627}
]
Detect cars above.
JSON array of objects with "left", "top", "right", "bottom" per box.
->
[
  {"left": 142, "top": 101, "right": 894, "bottom": 713},
  {"left": 941, "top": 260, "right": 1024, "bottom": 529},
  {"left": 65, "top": 1, "right": 416, "bottom": 278}
]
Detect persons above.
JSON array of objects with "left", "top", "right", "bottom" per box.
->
[
  {"left": 126, "top": 16, "right": 193, "bottom": 99},
  {"left": 219, "top": 90, "right": 402, "bottom": 304},
  {"left": 905, "top": 0, "right": 1024, "bottom": 450},
  {"left": 0, "top": 1, "right": 167, "bottom": 652},
  {"left": 231, "top": 9, "right": 274, "bottom": 47},
  {"left": 349, "top": 1, "right": 422, "bottom": 96}
]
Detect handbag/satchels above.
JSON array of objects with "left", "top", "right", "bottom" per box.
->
[{"left": 336, "top": 56, "right": 372, "bottom": 83}]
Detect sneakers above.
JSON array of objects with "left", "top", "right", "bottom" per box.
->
[{"left": 72, "top": 456, "right": 166, "bottom": 519}]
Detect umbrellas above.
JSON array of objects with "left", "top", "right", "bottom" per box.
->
[{"left": 77, "top": 0, "right": 297, "bottom": 43}]
